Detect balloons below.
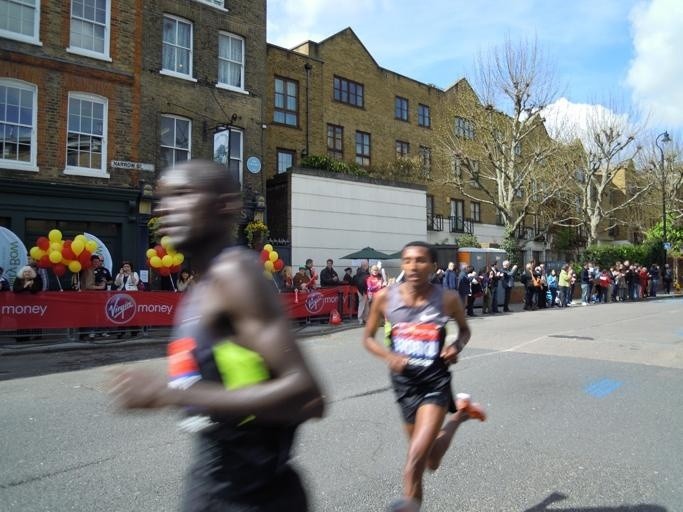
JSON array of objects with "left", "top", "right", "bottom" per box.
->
[
  {"left": 146, "top": 236, "right": 184, "bottom": 277},
  {"left": 260, "top": 244, "right": 285, "bottom": 274},
  {"left": 30, "top": 228, "right": 97, "bottom": 277}
]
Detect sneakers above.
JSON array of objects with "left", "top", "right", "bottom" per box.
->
[
  {"left": 80, "top": 333, "right": 111, "bottom": 343},
  {"left": 454, "top": 392, "right": 486, "bottom": 423}
]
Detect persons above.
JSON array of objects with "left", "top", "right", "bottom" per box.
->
[
  {"left": 276, "top": 259, "right": 388, "bottom": 324},
  {"left": 363, "top": 241, "right": 487, "bottom": 512},
  {"left": 0, "top": 254, "right": 48, "bottom": 342},
  {"left": 430, "top": 254, "right": 673, "bottom": 317},
  {"left": 107, "top": 157, "right": 324, "bottom": 512},
  {"left": 72, "top": 255, "right": 200, "bottom": 342}
]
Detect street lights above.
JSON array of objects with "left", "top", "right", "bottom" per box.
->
[{"left": 654, "top": 131, "right": 671, "bottom": 270}]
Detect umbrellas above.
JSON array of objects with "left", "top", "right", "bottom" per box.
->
[
  {"left": 340, "top": 247, "right": 393, "bottom": 267},
  {"left": 389, "top": 252, "right": 401, "bottom": 259}
]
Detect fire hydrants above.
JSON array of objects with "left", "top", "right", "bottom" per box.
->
[{"left": 675, "top": 284, "right": 681, "bottom": 293}]
